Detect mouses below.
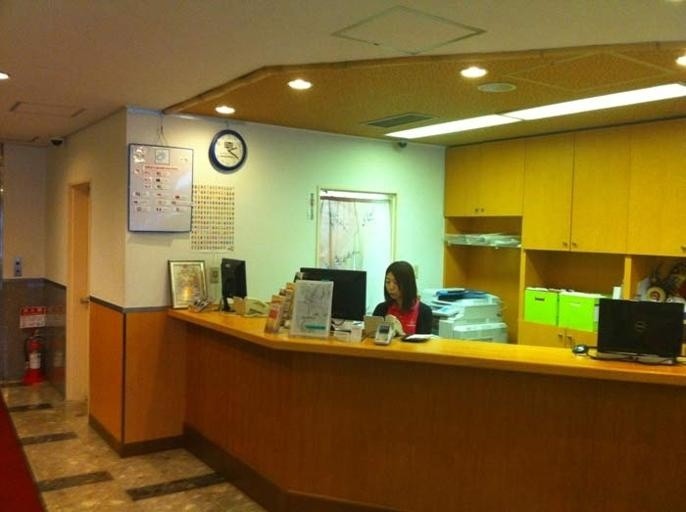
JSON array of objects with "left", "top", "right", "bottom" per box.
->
[{"left": 573, "top": 345, "right": 586, "bottom": 354}]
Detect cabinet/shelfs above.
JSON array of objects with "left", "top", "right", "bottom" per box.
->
[
  {"left": 443, "top": 137, "right": 526, "bottom": 344},
  {"left": 518, "top": 124, "right": 624, "bottom": 348},
  {"left": 625, "top": 116, "right": 686, "bottom": 307}
]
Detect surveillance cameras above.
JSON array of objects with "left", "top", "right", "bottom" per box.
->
[
  {"left": 398, "top": 141, "right": 407, "bottom": 147},
  {"left": 52, "top": 137, "right": 64, "bottom": 146}
]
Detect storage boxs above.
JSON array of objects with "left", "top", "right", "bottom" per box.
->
[
  {"left": 559, "top": 293, "right": 613, "bottom": 332},
  {"left": 524, "top": 288, "right": 558, "bottom": 326}
]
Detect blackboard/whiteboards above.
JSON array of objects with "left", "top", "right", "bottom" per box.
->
[{"left": 129, "top": 143, "right": 193, "bottom": 232}]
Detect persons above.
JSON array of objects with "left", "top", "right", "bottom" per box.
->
[{"left": 372, "top": 261, "right": 432, "bottom": 335}]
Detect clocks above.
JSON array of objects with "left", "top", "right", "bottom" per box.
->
[{"left": 211, "top": 129, "right": 245, "bottom": 170}]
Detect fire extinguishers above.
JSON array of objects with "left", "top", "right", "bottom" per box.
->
[{"left": 23, "top": 328, "right": 47, "bottom": 386}]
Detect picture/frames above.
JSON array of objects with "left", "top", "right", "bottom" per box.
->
[
  {"left": 288, "top": 279, "right": 335, "bottom": 339},
  {"left": 167, "top": 258, "right": 206, "bottom": 310}
]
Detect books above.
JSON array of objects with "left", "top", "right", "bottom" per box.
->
[{"left": 447, "top": 233, "right": 519, "bottom": 249}]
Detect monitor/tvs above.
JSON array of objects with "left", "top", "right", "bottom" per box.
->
[
  {"left": 596, "top": 297, "right": 685, "bottom": 358},
  {"left": 219, "top": 258, "right": 247, "bottom": 311},
  {"left": 300, "top": 267, "right": 367, "bottom": 321}
]
